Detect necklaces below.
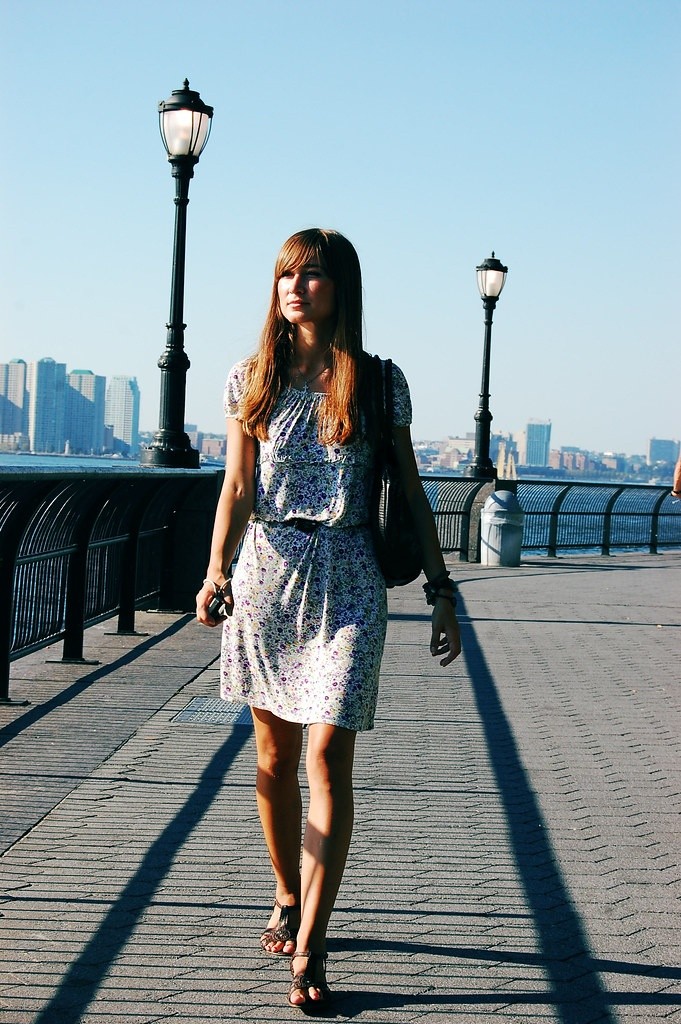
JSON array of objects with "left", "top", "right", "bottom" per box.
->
[{"left": 293, "top": 357, "right": 332, "bottom": 392}]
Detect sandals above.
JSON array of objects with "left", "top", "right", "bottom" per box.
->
[
  {"left": 286, "top": 951, "right": 332, "bottom": 1008},
  {"left": 261, "top": 897, "right": 301, "bottom": 956}
]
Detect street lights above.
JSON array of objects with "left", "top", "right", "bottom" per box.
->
[
  {"left": 463, "top": 250, "right": 508, "bottom": 478},
  {"left": 142, "top": 79, "right": 213, "bottom": 467}
]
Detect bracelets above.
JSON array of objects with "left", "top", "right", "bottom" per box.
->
[
  {"left": 673, "top": 491, "right": 681, "bottom": 494},
  {"left": 422, "top": 571, "right": 458, "bottom": 607}
]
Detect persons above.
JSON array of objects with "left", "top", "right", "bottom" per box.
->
[
  {"left": 196, "top": 229, "right": 462, "bottom": 1006},
  {"left": 671, "top": 459, "right": 681, "bottom": 498}
]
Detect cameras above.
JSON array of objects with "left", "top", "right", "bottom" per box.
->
[{"left": 208, "top": 596, "right": 227, "bottom": 622}]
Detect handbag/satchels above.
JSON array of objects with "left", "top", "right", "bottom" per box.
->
[{"left": 370, "top": 355, "right": 422, "bottom": 588}]
